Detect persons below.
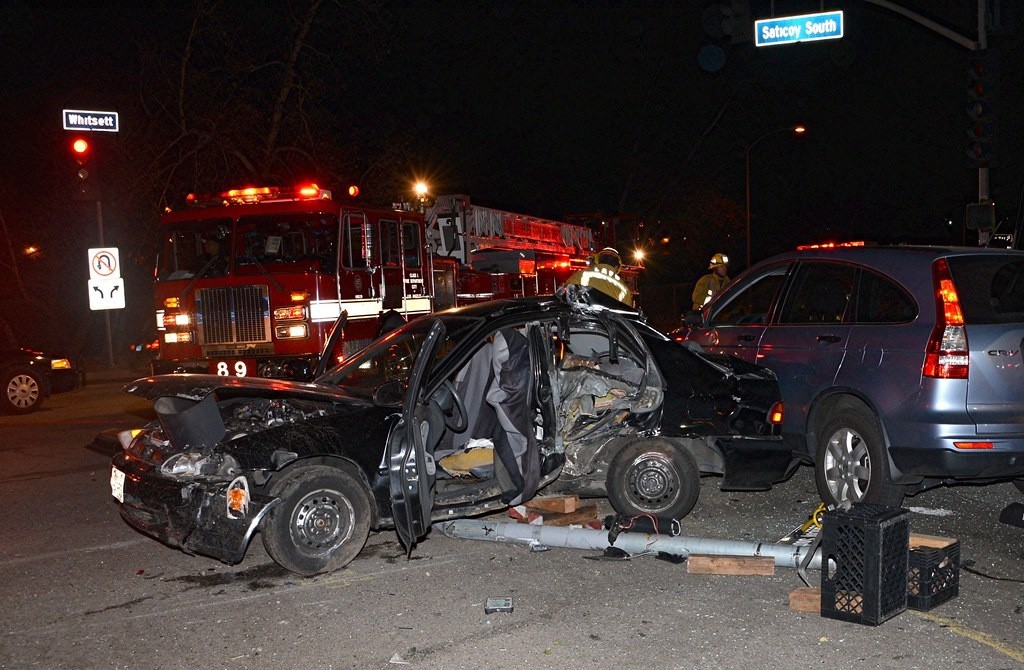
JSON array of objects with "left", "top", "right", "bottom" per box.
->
[
  {"left": 691, "top": 252, "right": 732, "bottom": 314},
  {"left": 193, "top": 233, "right": 229, "bottom": 276},
  {"left": 566, "top": 246, "right": 634, "bottom": 308}
]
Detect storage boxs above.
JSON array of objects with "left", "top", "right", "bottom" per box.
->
[
  {"left": 820, "top": 502, "right": 909, "bottom": 627},
  {"left": 908, "top": 539, "right": 961, "bottom": 612}
]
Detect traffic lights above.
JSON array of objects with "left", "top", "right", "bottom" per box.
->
[{"left": 63, "top": 131, "right": 93, "bottom": 203}]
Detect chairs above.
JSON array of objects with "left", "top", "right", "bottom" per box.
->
[{"left": 806, "top": 274, "right": 843, "bottom": 327}]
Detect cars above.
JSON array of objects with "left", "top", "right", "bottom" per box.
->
[
  {"left": 664, "top": 245, "right": 1020, "bottom": 527},
  {"left": 106, "top": 293, "right": 786, "bottom": 577},
  {"left": 0, "top": 341, "right": 87, "bottom": 415}
]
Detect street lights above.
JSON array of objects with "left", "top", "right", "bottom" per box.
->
[{"left": 742, "top": 124, "right": 806, "bottom": 249}]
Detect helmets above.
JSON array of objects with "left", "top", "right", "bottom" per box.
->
[
  {"left": 594, "top": 246, "right": 622, "bottom": 275},
  {"left": 709, "top": 253, "right": 728, "bottom": 269}
]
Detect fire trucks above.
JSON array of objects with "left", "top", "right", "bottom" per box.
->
[{"left": 152, "top": 179, "right": 608, "bottom": 386}]
